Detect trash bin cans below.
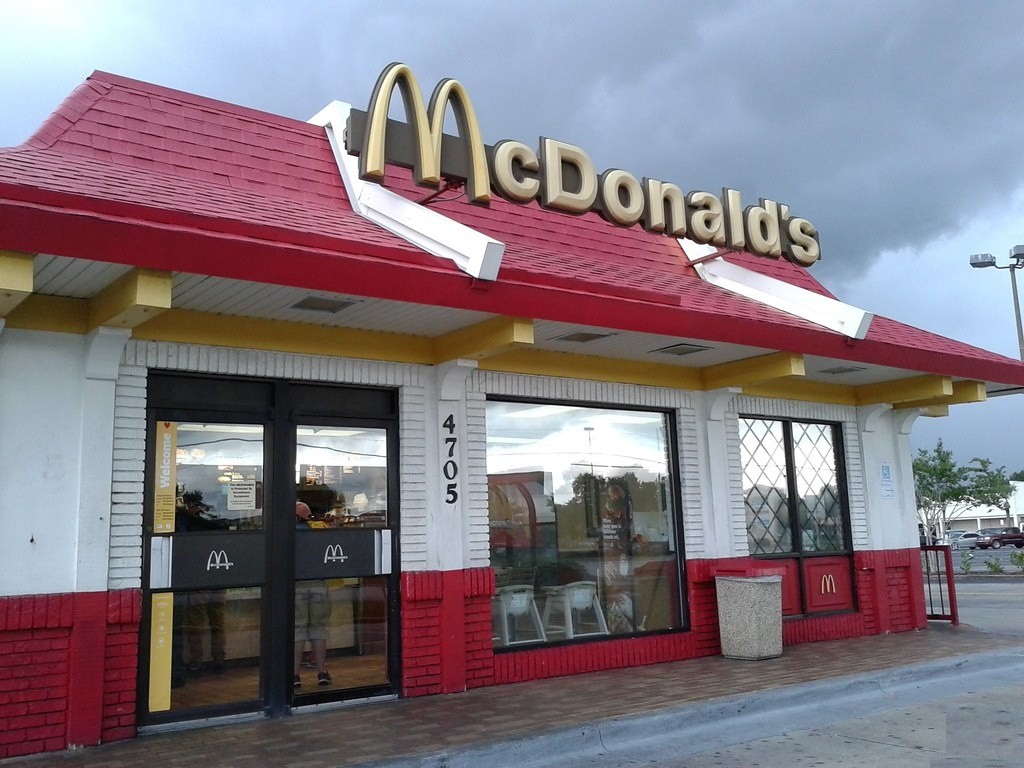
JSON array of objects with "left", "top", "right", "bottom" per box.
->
[{"left": 715, "top": 575, "right": 785, "bottom": 661}]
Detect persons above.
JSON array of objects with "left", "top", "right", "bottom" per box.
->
[
  {"left": 183, "top": 489, "right": 228, "bottom": 674},
  {"left": 171, "top": 485, "right": 187, "bottom": 689},
  {"left": 294, "top": 501, "right": 333, "bottom": 688}
]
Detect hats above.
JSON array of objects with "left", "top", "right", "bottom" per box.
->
[{"left": 296, "top": 501, "right": 312, "bottom": 520}]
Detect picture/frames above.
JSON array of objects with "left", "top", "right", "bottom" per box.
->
[{"left": 584, "top": 472, "right": 599, "bottom": 538}]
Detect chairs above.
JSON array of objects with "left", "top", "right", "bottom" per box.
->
[
  {"left": 493, "top": 584, "right": 548, "bottom": 646},
  {"left": 507, "top": 567, "right": 537, "bottom": 586},
  {"left": 541, "top": 580, "right": 611, "bottom": 640}
]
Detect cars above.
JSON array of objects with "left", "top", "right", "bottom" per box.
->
[
  {"left": 976, "top": 527, "right": 1024, "bottom": 549},
  {"left": 939, "top": 530, "right": 967, "bottom": 539},
  {"left": 920, "top": 536, "right": 942, "bottom": 546},
  {"left": 936, "top": 532, "right": 981, "bottom": 550}
]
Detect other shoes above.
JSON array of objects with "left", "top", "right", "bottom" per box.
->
[
  {"left": 190, "top": 664, "right": 201, "bottom": 671},
  {"left": 294, "top": 674, "right": 301, "bottom": 687},
  {"left": 215, "top": 667, "right": 224, "bottom": 675},
  {"left": 318, "top": 669, "right": 331, "bottom": 684}
]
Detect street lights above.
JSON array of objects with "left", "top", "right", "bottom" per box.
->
[
  {"left": 969, "top": 244, "right": 1024, "bottom": 360},
  {"left": 584, "top": 427, "right": 598, "bottom": 528}
]
